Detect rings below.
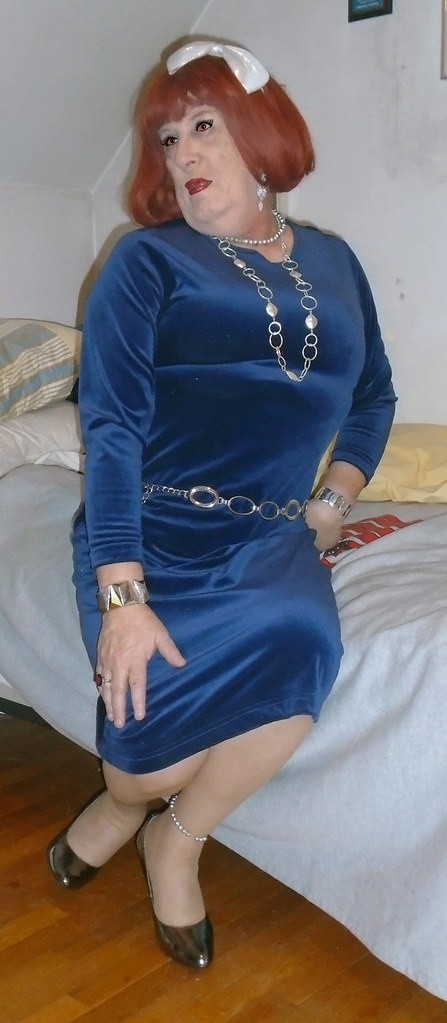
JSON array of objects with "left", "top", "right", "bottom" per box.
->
[{"left": 95, "top": 673, "right": 111, "bottom": 686}]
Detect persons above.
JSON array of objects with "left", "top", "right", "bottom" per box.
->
[{"left": 49, "top": 38, "right": 397, "bottom": 971}]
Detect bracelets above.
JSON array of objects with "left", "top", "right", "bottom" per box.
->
[
  {"left": 314, "top": 486, "right": 352, "bottom": 518},
  {"left": 96, "top": 578, "right": 150, "bottom": 612}
]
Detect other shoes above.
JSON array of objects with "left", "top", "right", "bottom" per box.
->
[
  {"left": 135, "top": 814, "right": 214, "bottom": 968},
  {"left": 48, "top": 790, "right": 152, "bottom": 889}
]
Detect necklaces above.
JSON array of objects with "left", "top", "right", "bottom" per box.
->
[{"left": 209, "top": 207, "right": 319, "bottom": 383}]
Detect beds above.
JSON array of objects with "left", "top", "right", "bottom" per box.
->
[{"left": 1, "top": 400, "right": 446, "bottom": 1004}]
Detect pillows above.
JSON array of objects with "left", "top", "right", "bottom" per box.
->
[
  {"left": 308, "top": 419, "right": 446, "bottom": 504},
  {"left": 1, "top": 313, "right": 81, "bottom": 424}
]
